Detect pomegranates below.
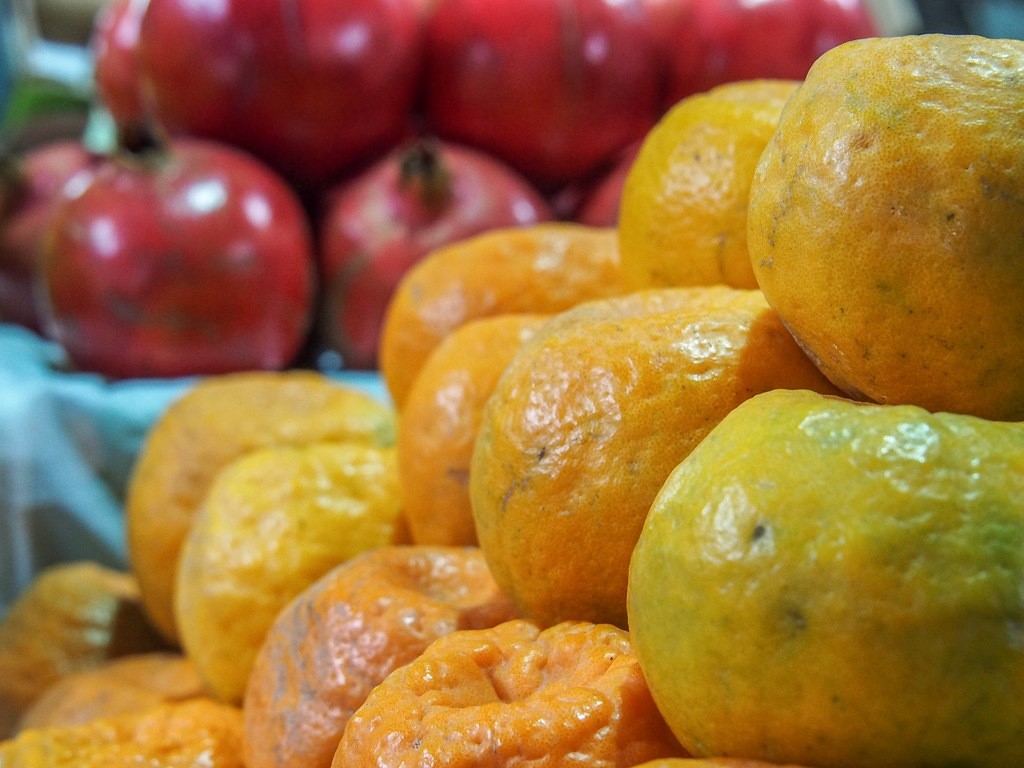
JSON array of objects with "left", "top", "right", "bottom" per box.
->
[{"left": 0, "top": 2, "right": 886, "bottom": 384}]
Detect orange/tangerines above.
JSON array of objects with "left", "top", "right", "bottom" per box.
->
[{"left": 0, "top": 33, "right": 1024, "bottom": 768}]
